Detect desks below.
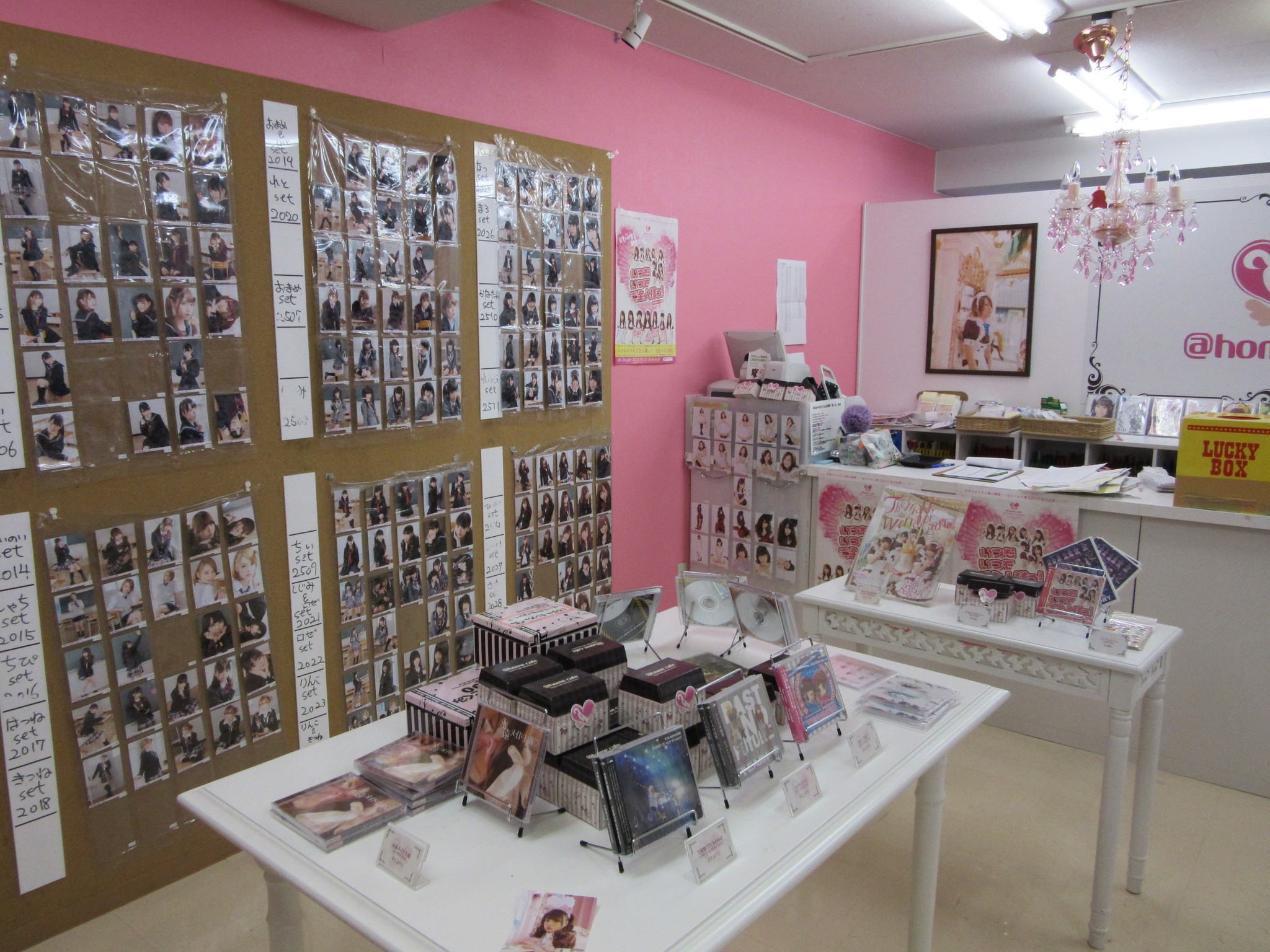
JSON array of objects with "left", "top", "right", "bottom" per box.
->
[
  {"left": 171, "top": 605, "right": 1009, "bottom": 951},
  {"left": 795, "top": 570, "right": 1181, "bottom": 951}
]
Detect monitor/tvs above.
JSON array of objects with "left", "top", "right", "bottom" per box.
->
[{"left": 723, "top": 329, "right": 786, "bottom": 379}]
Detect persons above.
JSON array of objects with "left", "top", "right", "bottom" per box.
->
[
  {"left": 0, "top": 87, "right": 1106, "bottom": 844},
  {"left": 515, "top": 908, "right": 575, "bottom": 952}
]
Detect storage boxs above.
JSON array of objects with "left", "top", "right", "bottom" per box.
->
[
  {"left": 1019, "top": 414, "right": 1117, "bottom": 441},
  {"left": 401, "top": 595, "right": 717, "bottom": 830},
  {"left": 954, "top": 409, "right": 1022, "bottom": 433}
]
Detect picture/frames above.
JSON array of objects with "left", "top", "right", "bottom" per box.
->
[{"left": 924, "top": 223, "right": 1042, "bottom": 377}]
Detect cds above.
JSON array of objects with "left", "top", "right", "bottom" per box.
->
[
  {"left": 733, "top": 591, "right": 784, "bottom": 642},
  {"left": 680, "top": 580, "right": 734, "bottom": 626},
  {"left": 597, "top": 598, "right": 650, "bottom": 642}
]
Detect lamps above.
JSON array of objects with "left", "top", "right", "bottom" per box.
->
[
  {"left": 946, "top": 0, "right": 1066, "bottom": 44},
  {"left": 1045, "top": 9, "right": 1201, "bottom": 287},
  {"left": 1063, "top": 84, "right": 1270, "bottom": 137},
  {"left": 1032, "top": 47, "right": 1162, "bottom": 121},
  {"left": 613, "top": 0, "right": 654, "bottom": 53}
]
